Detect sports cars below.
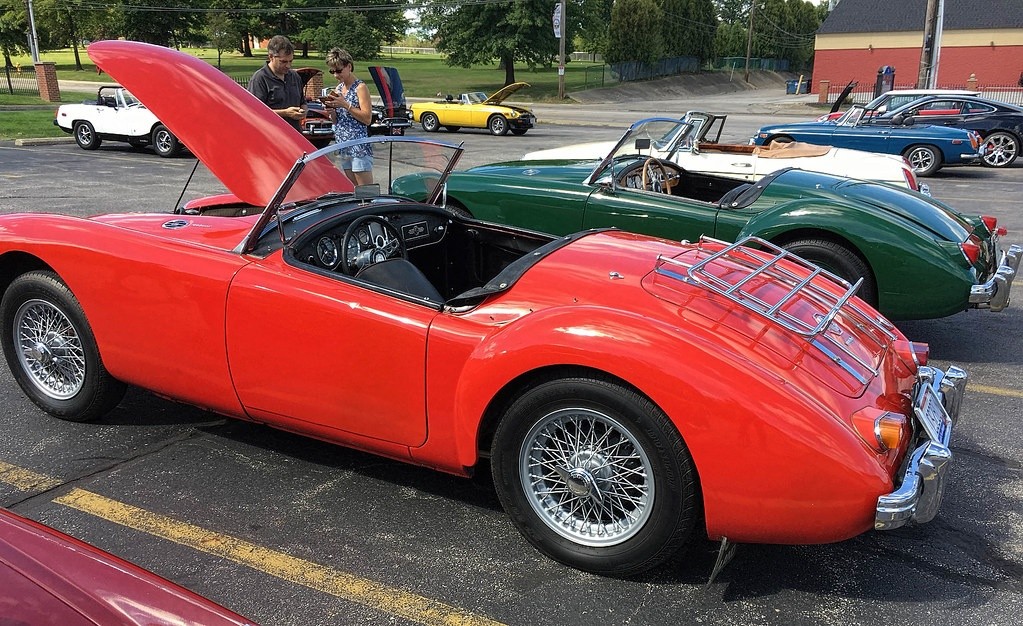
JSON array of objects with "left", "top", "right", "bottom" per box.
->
[
  {"left": 0, "top": 40, "right": 967, "bottom": 583},
  {"left": 390, "top": 118, "right": 1023, "bottom": 323},
  {"left": 409, "top": 82, "right": 538, "bottom": 136},
  {"left": 54, "top": 82, "right": 189, "bottom": 160},
  {"left": 518, "top": 110, "right": 930, "bottom": 199},
  {"left": 748, "top": 104, "right": 988, "bottom": 177}
]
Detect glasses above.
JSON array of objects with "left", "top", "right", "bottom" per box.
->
[{"left": 330, "top": 63, "right": 348, "bottom": 74}]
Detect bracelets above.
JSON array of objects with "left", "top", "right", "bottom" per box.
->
[{"left": 346, "top": 103, "right": 352, "bottom": 112}]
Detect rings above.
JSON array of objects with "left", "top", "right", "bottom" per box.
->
[{"left": 333, "top": 104, "right": 334, "bottom": 107}]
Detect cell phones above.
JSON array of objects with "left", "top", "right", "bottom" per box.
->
[
  {"left": 298, "top": 109, "right": 305, "bottom": 113},
  {"left": 319, "top": 90, "right": 339, "bottom": 105}
]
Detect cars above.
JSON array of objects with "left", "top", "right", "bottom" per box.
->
[
  {"left": 365, "top": 66, "right": 415, "bottom": 137},
  {"left": 291, "top": 66, "right": 335, "bottom": 149},
  {"left": 816, "top": 89, "right": 1023, "bottom": 169},
  {"left": 0, "top": 506, "right": 263, "bottom": 625}
]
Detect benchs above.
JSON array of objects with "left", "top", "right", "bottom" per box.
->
[{"left": 713, "top": 182, "right": 754, "bottom": 205}]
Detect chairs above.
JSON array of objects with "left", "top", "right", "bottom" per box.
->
[
  {"left": 457, "top": 94, "right": 466, "bottom": 103},
  {"left": 356, "top": 258, "right": 445, "bottom": 304},
  {"left": 446, "top": 94, "right": 454, "bottom": 105},
  {"left": 102, "top": 96, "right": 116, "bottom": 107},
  {"left": 891, "top": 114, "right": 904, "bottom": 125},
  {"left": 903, "top": 117, "right": 914, "bottom": 125}
]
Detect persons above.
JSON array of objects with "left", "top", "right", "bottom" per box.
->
[
  {"left": 247, "top": 35, "right": 308, "bottom": 136},
  {"left": 323, "top": 47, "right": 374, "bottom": 187}
]
